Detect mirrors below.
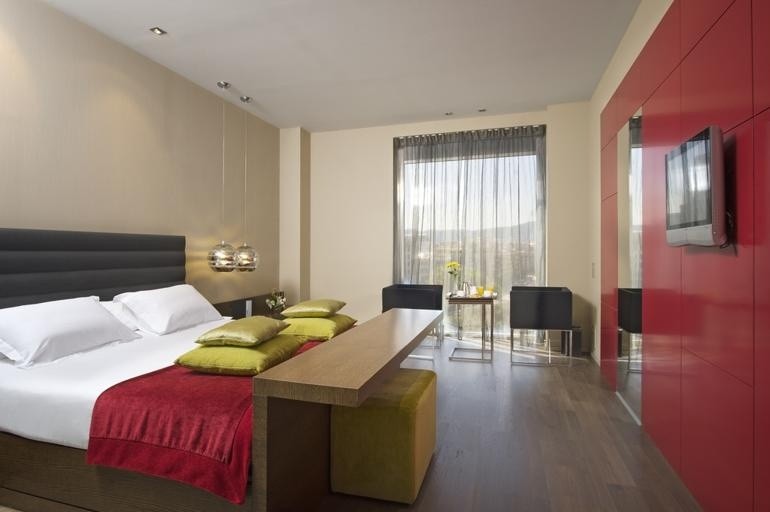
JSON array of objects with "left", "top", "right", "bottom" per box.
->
[{"left": 614, "top": 105, "right": 644, "bottom": 428}]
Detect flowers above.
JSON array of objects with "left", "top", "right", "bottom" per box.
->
[
  {"left": 264, "top": 288, "right": 287, "bottom": 312},
  {"left": 447, "top": 260, "right": 461, "bottom": 280}
]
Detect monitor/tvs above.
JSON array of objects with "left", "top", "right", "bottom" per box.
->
[{"left": 664, "top": 124, "right": 729, "bottom": 248}]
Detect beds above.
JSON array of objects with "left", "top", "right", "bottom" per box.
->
[{"left": 0, "top": 227, "right": 445, "bottom": 512}]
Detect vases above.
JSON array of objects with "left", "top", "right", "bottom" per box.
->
[{"left": 450, "top": 277, "right": 457, "bottom": 294}]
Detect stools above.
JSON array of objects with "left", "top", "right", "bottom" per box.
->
[{"left": 329, "top": 367, "right": 438, "bottom": 504}]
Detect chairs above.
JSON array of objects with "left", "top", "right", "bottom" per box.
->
[
  {"left": 619, "top": 286, "right": 641, "bottom": 374},
  {"left": 511, "top": 286, "right": 573, "bottom": 369},
  {"left": 380, "top": 283, "right": 443, "bottom": 360}
]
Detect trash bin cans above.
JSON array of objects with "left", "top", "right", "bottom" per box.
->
[{"left": 561, "top": 331, "right": 582, "bottom": 355}]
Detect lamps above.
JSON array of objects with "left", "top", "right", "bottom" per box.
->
[
  {"left": 207, "top": 80, "right": 233, "bottom": 274},
  {"left": 233, "top": 92, "right": 259, "bottom": 272}
]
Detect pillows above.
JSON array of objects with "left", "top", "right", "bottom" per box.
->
[
  {"left": 0, "top": 293, "right": 141, "bottom": 366},
  {"left": 194, "top": 315, "right": 291, "bottom": 346},
  {"left": 111, "top": 283, "right": 224, "bottom": 336},
  {"left": 174, "top": 334, "right": 311, "bottom": 375},
  {"left": 280, "top": 314, "right": 358, "bottom": 342},
  {"left": 278, "top": 298, "right": 348, "bottom": 318}
]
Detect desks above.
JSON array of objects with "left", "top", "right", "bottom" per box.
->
[
  {"left": 253, "top": 308, "right": 444, "bottom": 511},
  {"left": 447, "top": 291, "right": 495, "bottom": 362}
]
